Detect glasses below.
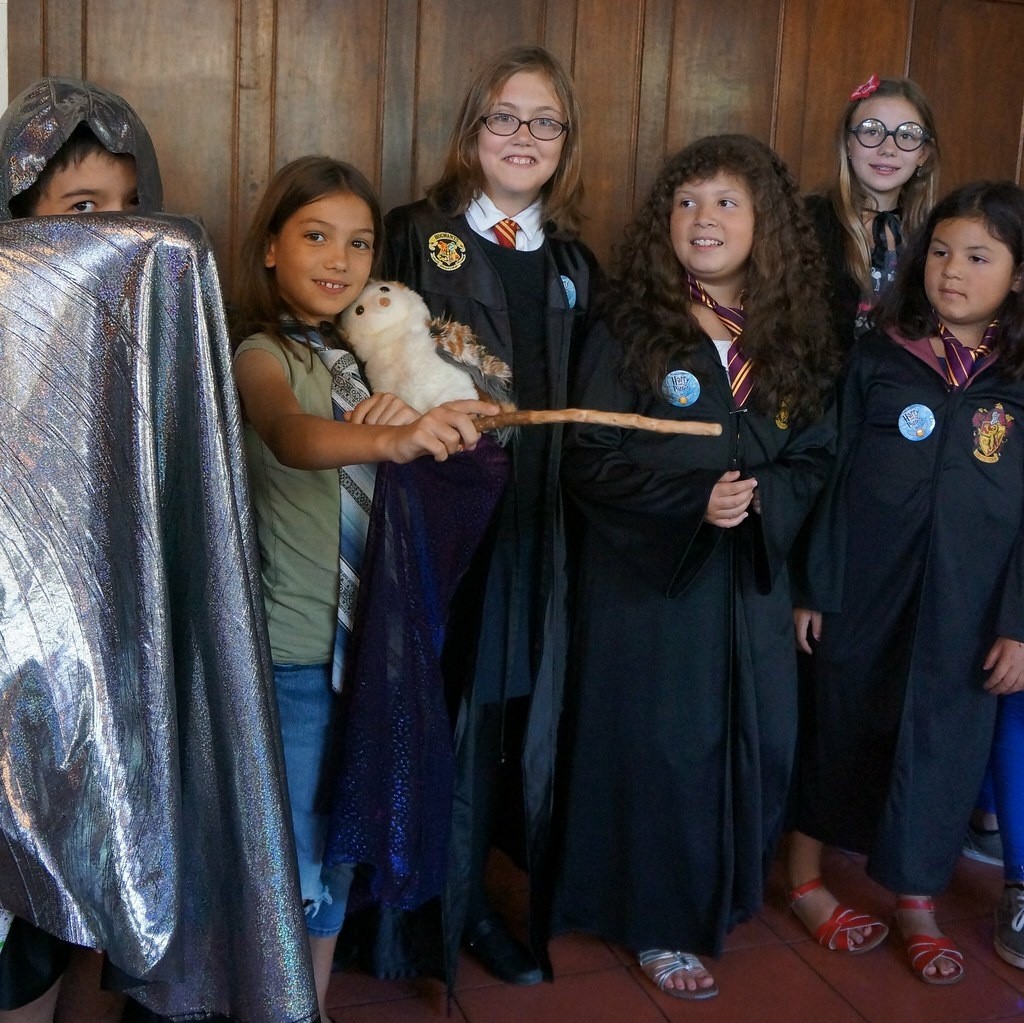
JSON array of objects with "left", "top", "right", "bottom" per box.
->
[
  {"left": 479, "top": 112, "right": 572, "bottom": 141},
  {"left": 849, "top": 118, "right": 931, "bottom": 152}
]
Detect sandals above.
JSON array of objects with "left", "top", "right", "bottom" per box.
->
[
  {"left": 786, "top": 877, "right": 888, "bottom": 955},
  {"left": 637, "top": 947, "right": 719, "bottom": 1001},
  {"left": 893, "top": 897, "right": 965, "bottom": 985}
]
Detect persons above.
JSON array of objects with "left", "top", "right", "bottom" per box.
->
[
  {"left": 762, "top": 67, "right": 950, "bottom": 893},
  {"left": 563, "top": 127, "right": 844, "bottom": 1009},
  {"left": 788, "top": 168, "right": 1024, "bottom": 989},
  {"left": 217, "top": 141, "right": 503, "bottom": 1022},
  {"left": 346, "top": 40, "right": 604, "bottom": 1012},
  {"left": 0, "top": 77, "right": 236, "bottom": 1023},
  {"left": 962, "top": 659, "right": 1024, "bottom": 970}
]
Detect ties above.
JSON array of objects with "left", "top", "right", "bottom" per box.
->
[
  {"left": 686, "top": 276, "right": 753, "bottom": 407},
  {"left": 278, "top": 318, "right": 376, "bottom": 693},
  {"left": 933, "top": 315, "right": 1000, "bottom": 385},
  {"left": 489, "top": 219, "right": 521, "bottom": 252}
]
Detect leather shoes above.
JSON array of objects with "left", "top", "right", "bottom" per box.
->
[
  {"left": 464, "top": 912, "right": 543, "bottom": 986},
  {"left": 3, "top": 918, "right": 72, "bottom": 1011}
]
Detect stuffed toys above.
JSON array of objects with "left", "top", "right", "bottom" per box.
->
[{"left": 334, "top": 270, "right": 525, "bottom": 449}]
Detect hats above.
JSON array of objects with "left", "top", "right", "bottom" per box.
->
[{"left": 2, "top": 79, "right": 163, "bottom": 217}]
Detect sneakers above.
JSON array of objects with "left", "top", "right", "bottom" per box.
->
[
  {"left": 963, "top": 826, "right": 1003, "bottom": 867},
  {"left": 995, "top": 888, "right": 1022, "bottom": 971}
]
